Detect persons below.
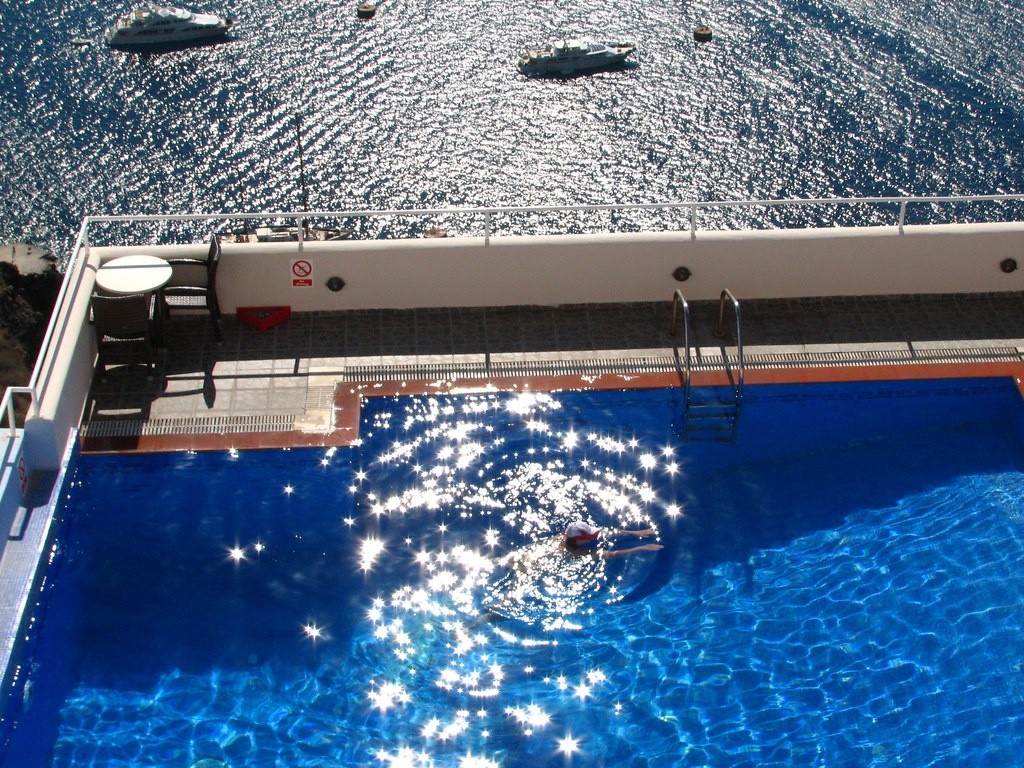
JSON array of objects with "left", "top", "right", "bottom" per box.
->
[{"left": 565, "top": 521, "right": 601, "bottom": 546}]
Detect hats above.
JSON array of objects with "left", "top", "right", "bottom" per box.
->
[{"left": 565, "top": 522, "right": 600, "bottom": 539}]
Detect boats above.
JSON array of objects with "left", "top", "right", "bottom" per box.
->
[
  {"left": 105, "top": 0, "right": 234, "bottom": 48},
  {"left": 514, "top": 37, "right": 637, "bottom": 76}
]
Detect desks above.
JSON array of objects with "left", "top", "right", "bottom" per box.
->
[{"left": 96, "top": 254, "right": 173, "bottom": 349}]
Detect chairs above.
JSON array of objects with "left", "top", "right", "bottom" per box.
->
[
  {"left": 91, "top": 294, "right": 159, "bottom": 386},
  {"left": 160, "top": 235, "right": 222, "bottom": 351}
]
